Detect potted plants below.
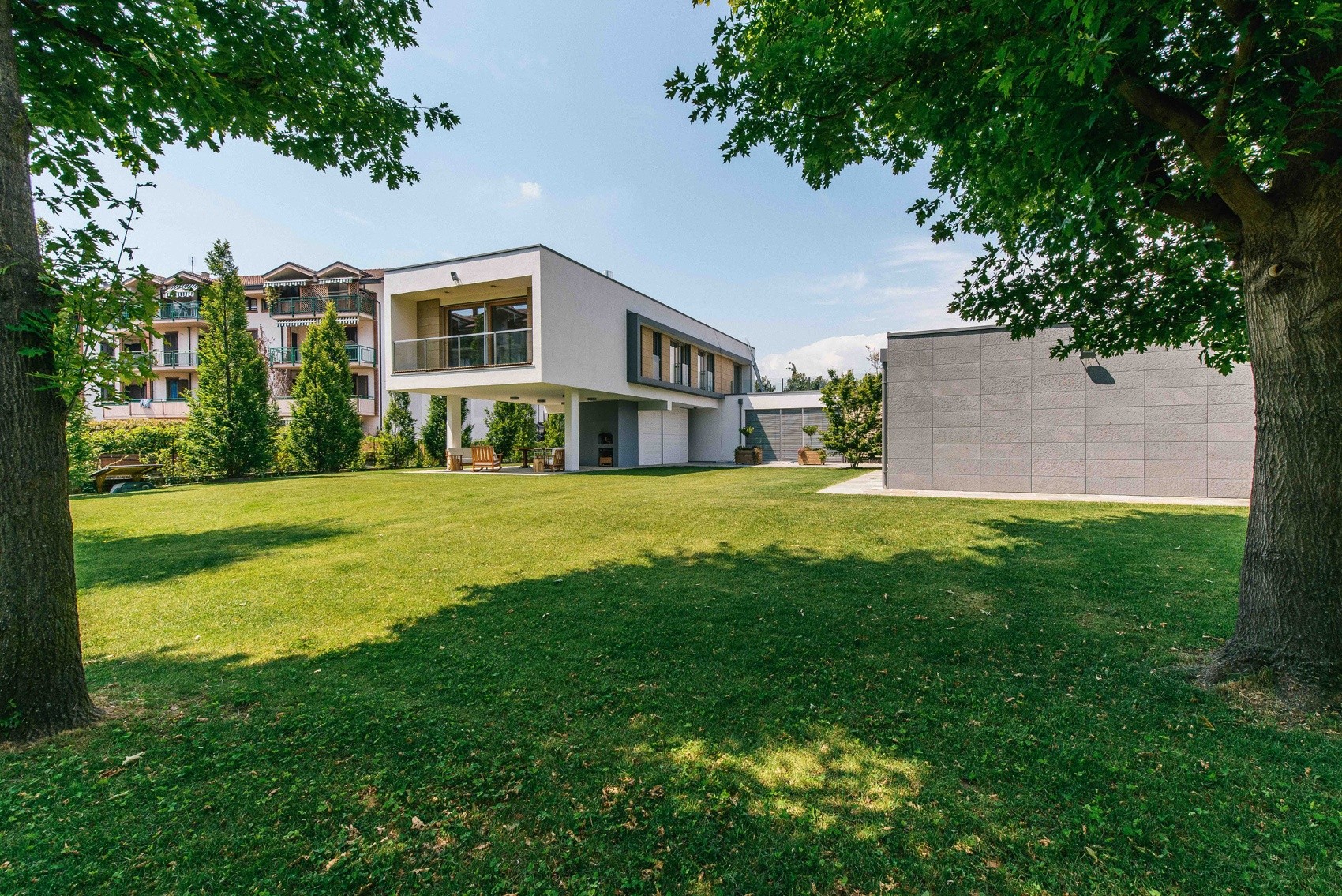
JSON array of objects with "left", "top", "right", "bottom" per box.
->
[
  {"left": 734, "top": 426, "right": 762, "bottom": 465},
  {"left": 798, "top": 425, "right": 826, "bottom": 465}
]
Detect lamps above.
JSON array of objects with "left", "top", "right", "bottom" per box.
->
[
  {"left": 1079, "top": 345, "right": 1096, "bottom": 359},
  {"left": 451, "top": 271, "right": 459, "bottom": 281}
]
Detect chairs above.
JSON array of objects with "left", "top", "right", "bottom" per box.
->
[
  {"left": 472, "top": 445, "right": 503, "bottom": 472},
  {"left": 532, "top": 447, "right": 552, "bottom": 461}
]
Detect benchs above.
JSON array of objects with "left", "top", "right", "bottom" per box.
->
[
  {"left": 535, "top": 447, "right": 565, "bottom": 471},
  {"left": 445, "top": 447, "right": 497, "bottom": 469}
]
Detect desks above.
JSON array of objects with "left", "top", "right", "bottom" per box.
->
[{"left": 516, "top": 447, "right": 533, "bottom": 468}]
